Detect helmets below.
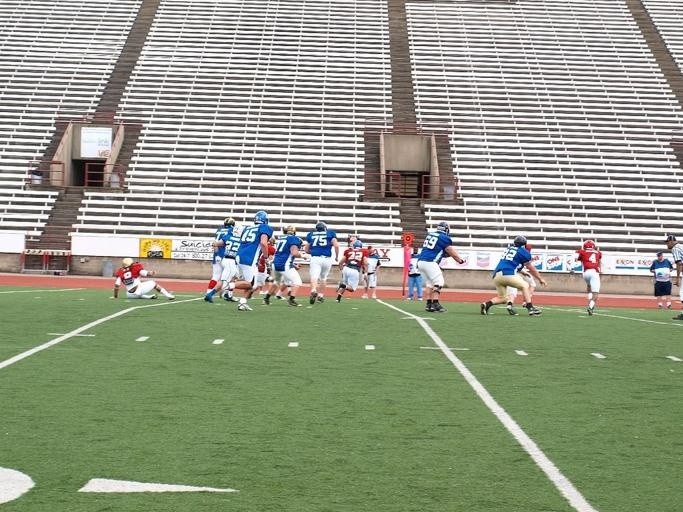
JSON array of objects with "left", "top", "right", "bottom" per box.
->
[
  {"left": 316, "top": 223, "right": 327, "bottom": 231},
  {"left": 583, "top": 240, "right": 595, "bottom": 249},
  {"left": 353, "top": 240, "right": 362, "bottom": 248},
  {"left": 122, "top": 258, "right": 133, "bottom": 267},
  {"left": 224, "top": 218, "right": 235, "bottom": 227},
  {"left": 514, "top": 236, "right": 531, "bottom": 251},
  {"left": 438, "top": 222, "right": 449, "bottom": 234},
  {"left": 287, "top": 225, "right": 296, "bottom": 234},
  {"left": 254, "top": 211, "right": 268, "bottom": 224}
]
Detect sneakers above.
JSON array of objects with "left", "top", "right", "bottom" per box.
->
[
  {"left": 169, "top": 295, "right": 176, "bottom": 300},
  {"left": 262, "top": 295, "right": 302, "bottom": 306},
  {"left": 672, "top": 313, "right": 683, "bottom": 320},
  {"left": 481, "top": 302, "right": 487, "bottom": 314},
  {"left": 151, "top": 294, "right": 157, "bottom": 299},
  {"left": 507, "top": 308, "right": 520, "bottom": 315},
  {"left": 522, "top": 302, "right": 542, "bottom": 315},
  {"left": 426, "top": 303, "right": 447, "bottom": 311},
  {"left": 205, "top": 293, "right": 253, "bottom": 311},
  {"left": 310, "top": 291, "right": 324, "bottom": 304},
  {"left": 588, "top": 308, "right": 592, "bottom": 315}
]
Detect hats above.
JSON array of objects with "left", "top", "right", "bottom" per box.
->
[{"left": 664, "top": 235, "right": 675, "bottom": 242}]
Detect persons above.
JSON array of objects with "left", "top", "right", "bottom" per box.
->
[
  {"left": 107, "top": 257, "right": 175, "bottom": 301},
  {"left": 203, "top": 208, "right": 382, "bottom": 312},
  {"left": 414, "top": 221, "right": 468, "bottom": 313},
  {"left": 662, "top": 234, "right": 682, "bottom": 321},
  {"left": 405, "top": 245, "right": 424, "bottom": 301},
  {"left": 649, "top": 250, "right": 674, "bottom": 311},
  {"left": 505, "top": 243, "right": 536, "bottom": 316},
  {"left": 572, "top": 239, "right": 602, "bottom": 316},
  {"left": 478, "top": 235, "right": 548, "bottom": 316}
]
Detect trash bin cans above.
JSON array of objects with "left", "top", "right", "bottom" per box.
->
[
  {"left": 110, "top": 173, "right": 119, "bottom": 187},
  {"left": 443, "top": 185, "right": 455, "bottom": 200},
  {"left": 102, "top": 260, "right": 112, "bottom": 277},
  {"left": 29, "top": 170, "right": 42, "bottom": 184}
]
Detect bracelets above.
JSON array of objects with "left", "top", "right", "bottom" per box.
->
[{"left": 458, "top": 259, "right": 463, "bottom": 264}]
